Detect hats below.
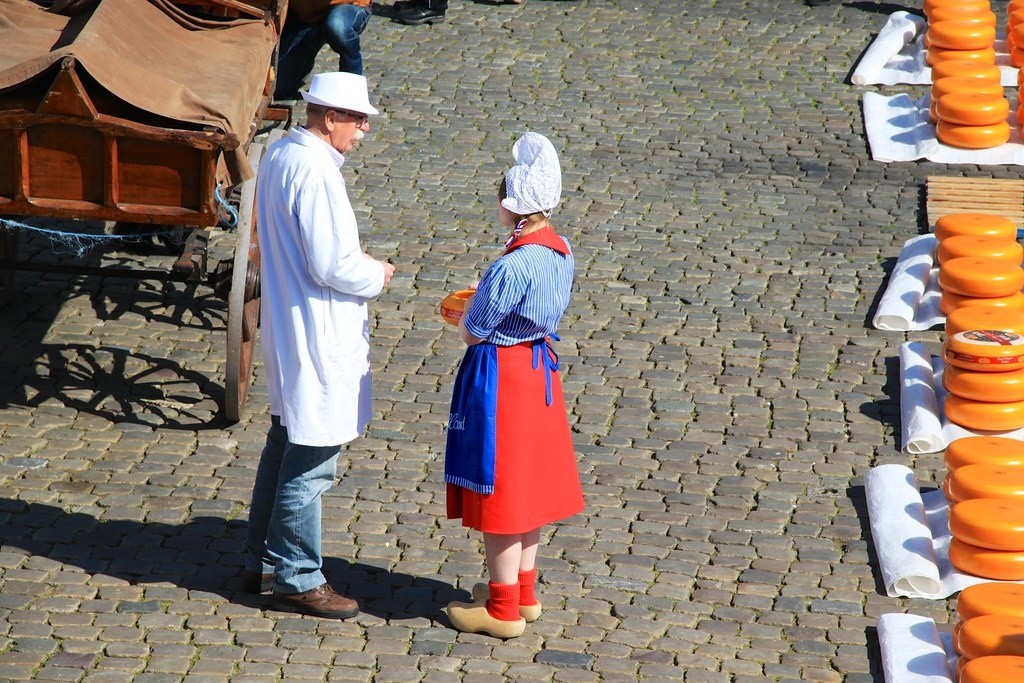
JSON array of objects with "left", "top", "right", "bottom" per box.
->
[
  {"left": 501, "top": 132, "right": 562, "bottom": 218},
  {"left": 301, "top": 72, "right": 379, "bottom": 115}
]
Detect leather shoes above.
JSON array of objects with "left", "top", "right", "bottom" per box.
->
[
  {"left": 397, "top": 7, "right": 445, "bottom": 24},
  {"left": 270, "top": 582, "right": 359, "bottom": 619},
  {"left": 254, "top": 571, "right": 275, "bottom": 591}
]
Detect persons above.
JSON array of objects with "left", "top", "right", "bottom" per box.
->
[
  {"left": 275, "top": 0, "right": 373, "bottom": 101},
  {"left": 447, "top": 130, "right": 587, "bottom": 638},
  {"left": 390, "top": 0, "right": 449, "bottom": 25},
  {"left": 243, "top": 72, "right": 396, "bottom": 619}
]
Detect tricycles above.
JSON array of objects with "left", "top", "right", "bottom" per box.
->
[{"left": 0, "top": 0, "right": 291, "bottom": 422}]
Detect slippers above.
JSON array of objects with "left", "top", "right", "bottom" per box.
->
[
  {"left": 472, "top": 582, "right": 542, "bottom": 622},
  {"left": 447, "top": 599, "right": 526, "bottom": 638}
]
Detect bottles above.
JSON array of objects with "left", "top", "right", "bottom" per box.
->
[{"left": 440, "top": 290, "right": 476, "bottom": 326}]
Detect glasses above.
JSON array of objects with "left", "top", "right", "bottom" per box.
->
[{"left": 326, "top": 108, "right": 368, "bottom": 128}]
